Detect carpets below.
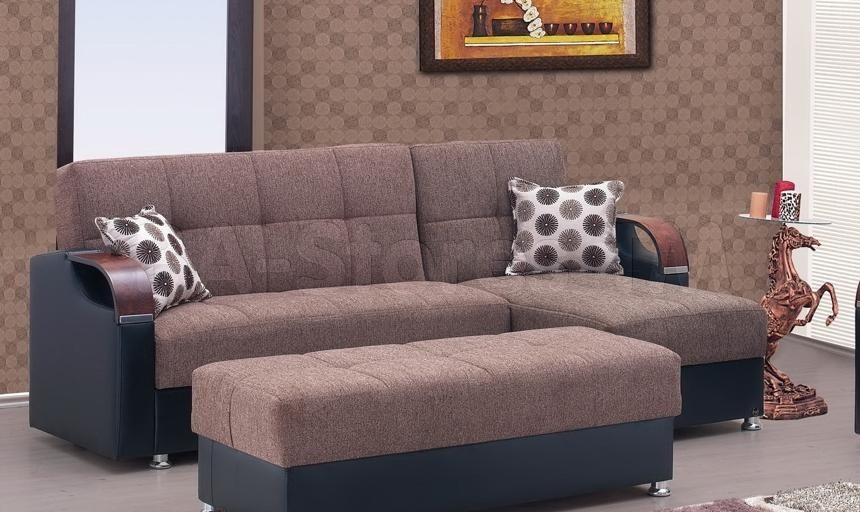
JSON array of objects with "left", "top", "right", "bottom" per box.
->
[{"left": 666, "top": 480, "right": 859, "bottom": 512}]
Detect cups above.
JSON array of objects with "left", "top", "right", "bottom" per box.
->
[{"left": 772, "top": 180, "right": 801, "bottom": 222}]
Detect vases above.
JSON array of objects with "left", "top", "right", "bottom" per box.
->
[{"left": 472, "top": 5, "right": 487, "bottom": 36}]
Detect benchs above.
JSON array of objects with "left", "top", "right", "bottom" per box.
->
[{"left": 184, "top": 322, "right": 682, "bottom": 510}]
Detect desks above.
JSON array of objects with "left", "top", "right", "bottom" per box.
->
[{"left": 736, "top": 209, "right": 840, "bottom": 424}]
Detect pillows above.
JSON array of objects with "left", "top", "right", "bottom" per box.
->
[
  {"left": 94, "top": 203, "right": 214, "bottom": 321},
  {"left": 500, "top": 176, "right": 625, "bottom": 279}
]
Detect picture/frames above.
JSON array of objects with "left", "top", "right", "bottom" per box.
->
[{"left": 418, "top": 0, "right": 652, "bottom": 79}]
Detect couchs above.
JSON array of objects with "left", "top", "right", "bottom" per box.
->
[{"left": 16, "top": 139, "right": 767, "bottom": 473}]
